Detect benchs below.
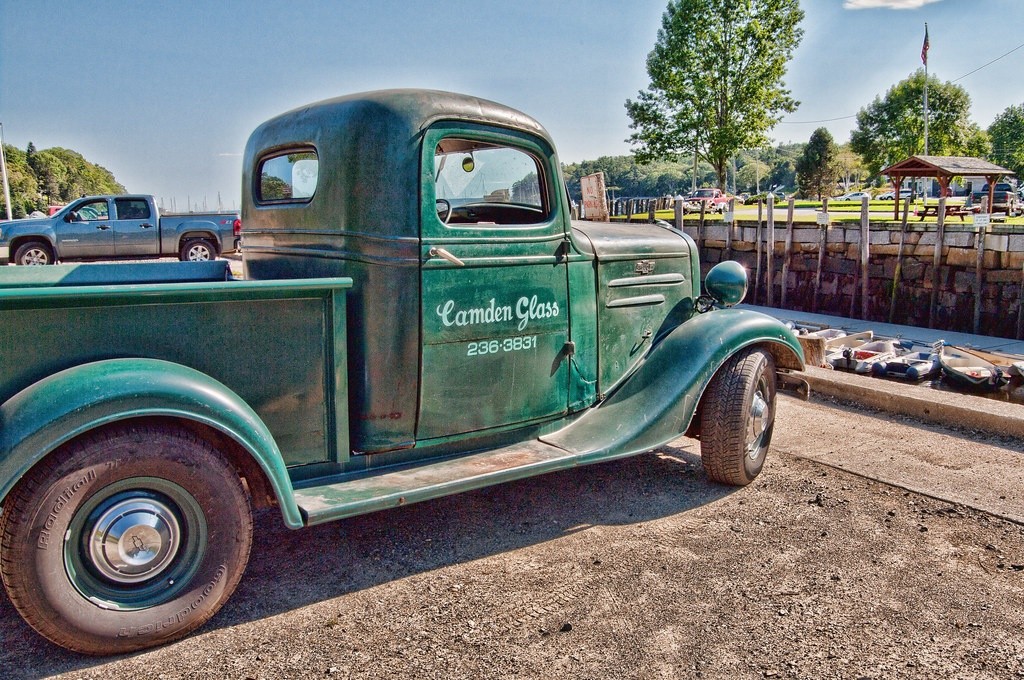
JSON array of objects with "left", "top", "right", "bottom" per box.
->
[{"left": 917, "top": 211, "right": 968, "bottom": 222}]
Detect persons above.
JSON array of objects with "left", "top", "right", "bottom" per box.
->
[{"left": 965, "top": 192, "right": 973, "bottom": 208}]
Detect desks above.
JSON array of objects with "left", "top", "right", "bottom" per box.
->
[{"left": 920, "top": 205, "right": 964, "bottom": 223}]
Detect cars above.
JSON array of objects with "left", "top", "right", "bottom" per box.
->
[
  {"left": 881, "top": 189, "right": 916, "bottom": 201},
  {"left": 685, "top": 189, "right": 726, "bottom": 213},
  {"left": 1017, "top": 182, "right": 1024, "bottom": 200},
  {"left": 841, "top": 192, "right": 869, "bottom": 201},
  {"left": 938, "top": 187, "right": 953, "bottom": 198},
  {"left": 774, "top": 192, "right": 785, "bottom": 200}
]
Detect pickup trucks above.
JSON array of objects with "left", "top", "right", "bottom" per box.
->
[
  {"left": 0, "top": 194, "right": 242, "bottom": 265},
  {"left": 0, "top": 84, "right": 807, "bottom": 655},
  {"left": 971, "top": 182, "right": 1019, "bottom": 216}
]
